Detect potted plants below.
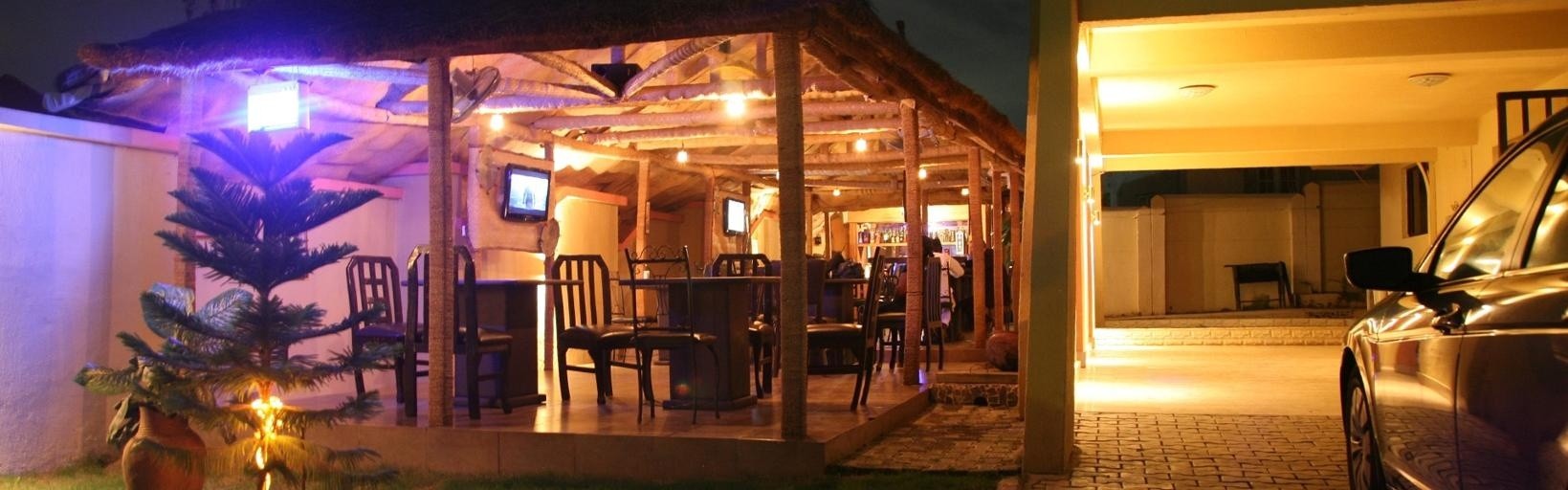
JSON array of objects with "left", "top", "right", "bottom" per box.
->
[{"left": 72, "top": 277, "right": 255, "bottom": 490}]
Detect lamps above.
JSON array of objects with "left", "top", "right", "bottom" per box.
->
[
  {"left": 1180, "top": 84, "right": 1215, "bottom": 99},
  {"left": 1409, "top": 74, "right": 1451, "bottom": 89}
]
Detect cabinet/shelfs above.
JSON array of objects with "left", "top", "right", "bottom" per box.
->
[{"left": 852, "top": 217, "right": 968, "bottom": 261}]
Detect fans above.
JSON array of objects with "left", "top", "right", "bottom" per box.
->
[
  {"left": 449, "top": 62, "right": 502, "bottom": 124},
  {"left": 883, "top": 126, "right": 940, "bottom": 154}
]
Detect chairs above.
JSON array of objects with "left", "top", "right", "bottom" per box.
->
[
  {"left": 808, "top": 246, "right": 944, "bottom": 412},
  {"left": 404, "top": 244, "right": 516, "bottom": 421},
  {"left": 344, "top": 252, "right": 432, "bottom": 403},
  {"left": 553, "top": 245, "right": 780, "bottom": 425}
]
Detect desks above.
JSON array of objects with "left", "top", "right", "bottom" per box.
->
[{"left": 399, "top": 279, "right": 585, "bottom": 413}]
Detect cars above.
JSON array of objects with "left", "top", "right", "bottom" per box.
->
[{"left": 1339, "top": 103, "right": 1568, "bottom": 490}]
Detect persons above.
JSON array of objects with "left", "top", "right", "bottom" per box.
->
[
  {"left": 848, "top": 235, "right": 934, "bottom": 370},
  {"left": 963, "top": 248, "right": 1011, "bottom": 333},
  {"left": 924, "top": 237, "right": 965, "bottom": 345}
]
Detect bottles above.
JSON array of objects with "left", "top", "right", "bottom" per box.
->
[
  {"left": 858, "top": 227, "right": 955, "bottom": 243},
  {"left": 643, "top": 262, "right": 651, "bottom": 280},
  {"left": 864, "top": 258, "right": 873, "bottom": 280}
]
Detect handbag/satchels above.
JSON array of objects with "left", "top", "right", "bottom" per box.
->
[{"left": 832, "top": 258, "right": 864, "bottom": 279}]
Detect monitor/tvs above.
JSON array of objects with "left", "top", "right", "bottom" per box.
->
[
  {"left": 503, "top": 163, "right": 551, "bottom": 222},
  {"left": 724, "top": 197, "right": 746, "bottom": 235}
]
